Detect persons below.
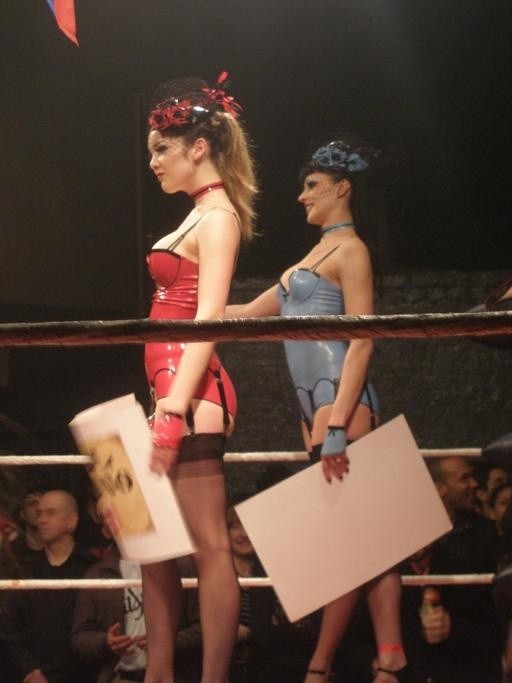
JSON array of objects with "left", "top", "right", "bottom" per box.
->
[
  {"left": 225, "top": 463, "right": 324, "bottom": 681},
  {"left": 3, "top": 451, "right": 203, "bottom": 681},
  {"left": 136, "top": 69, "right": 261, "bottom": 682},
  {"left": 329, "top": 457, "right": 508, "bottom": 681},
  {"left": 220, "top": 138, "right": 413, "bottom": 683}
]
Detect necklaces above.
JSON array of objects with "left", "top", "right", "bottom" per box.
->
[
  {"left": 190, "top": 180, "right": 225, "bottom": 201},
  {"left": 319, "top": 222, "right": 358, "bottom": 234}
]
